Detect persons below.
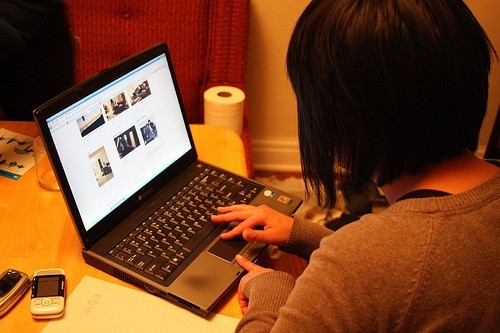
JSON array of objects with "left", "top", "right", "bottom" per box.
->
[
  {"left": 119, "top": 139, "right": 134, "bottom": 158},
  {"left": 211, "top": 0, "right": 500, "bottom": 333},
  {"left": 143, "top": 119, "right": 156, "bottom": 145},
  {"left": 131, "top": 81, "right": 148, "bottom": 101},
  {"left": 0, "top": 0, "right": 72, "bottom": 120}
]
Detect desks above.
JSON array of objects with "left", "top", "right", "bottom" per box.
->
[{"left": 0, "top": 120, "right": 248, "bottom": 333}]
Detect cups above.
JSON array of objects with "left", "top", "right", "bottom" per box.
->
[{"left": 33, "top": 136, "right": 61, "bottom": 191}]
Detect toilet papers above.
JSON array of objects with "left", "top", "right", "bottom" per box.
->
[{"left": 203, "top": 85, "right": 246, "bottom": 136}]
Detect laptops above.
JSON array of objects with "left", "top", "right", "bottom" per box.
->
[{"left": 34, "top": 41, "right": 304, "bottom": 318}]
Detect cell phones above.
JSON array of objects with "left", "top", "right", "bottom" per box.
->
[
  {"left": 31, "top": 268, "right": 66, "bottom": 319},
  {"left": 0, "top": 268, "right": 34, "bottom": 318}
]
